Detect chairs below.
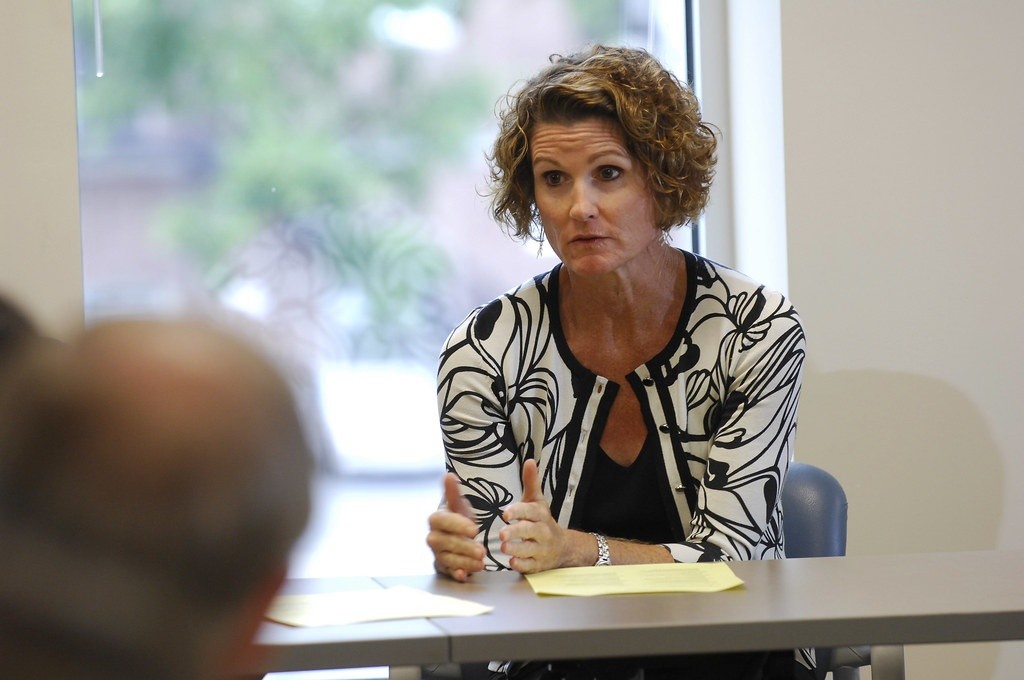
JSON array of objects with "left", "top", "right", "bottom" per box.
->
[{"left": 780, "top": 464, "right": 907, "bottom": 679}]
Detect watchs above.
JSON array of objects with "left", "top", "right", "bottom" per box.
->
[{"left": 587, "top": 532, "right": 612, "bottom": 567}]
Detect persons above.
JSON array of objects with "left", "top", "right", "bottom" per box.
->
[
  {"left": 427, "top": 43, "right": 816, "bottom": 680},
  {"left": 0, "top": 289, "right": 316, "bottom": 680}
]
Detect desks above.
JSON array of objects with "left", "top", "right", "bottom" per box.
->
[
  {"left": 373, "top": 548, "right": 1024, "bottom": 680},
  {"left": 230, "top": 571, "right": 451, "bottom": 677}
]
